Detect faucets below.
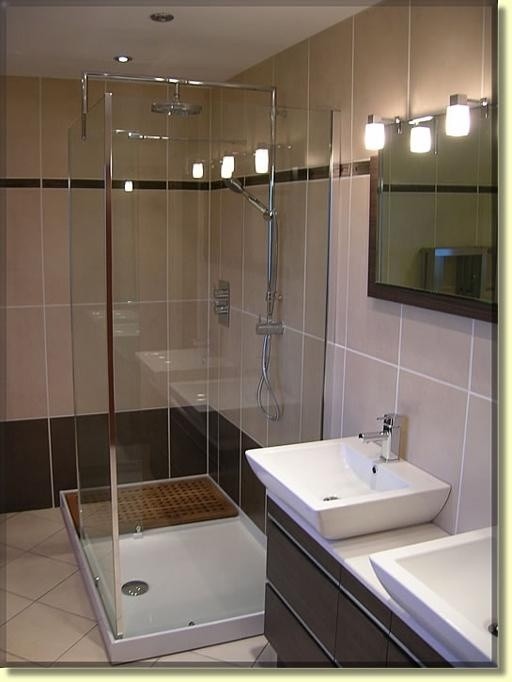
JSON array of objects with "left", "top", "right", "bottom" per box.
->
[
  {"left": 192, "top": 337, "right": 216, "bottom": 361},
  {"left": 357, "top": 412, "right": 400, "bottom": 461}
]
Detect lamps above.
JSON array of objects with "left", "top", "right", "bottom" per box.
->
[
  {"left": 221, "top": 156, "right": 235, "bottom": 179},
  {"left": 193, "top": 162, "right": 203, "bottom": 179},
  {"left": 408, "top": 115, "right": 433, "bottom": 153},
  {"left": 443, "top": 93, "right": 489, "bottom": 138},
  {"left": 363, "top": 113, "right": 402, "bottom": 154},
  {"left": 255, "top": 150, "right": 268, "bottom": 174}
]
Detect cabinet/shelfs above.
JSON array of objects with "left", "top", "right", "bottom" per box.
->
[{"left": 264, "top": 494, "right": 457, "bottom": 667}]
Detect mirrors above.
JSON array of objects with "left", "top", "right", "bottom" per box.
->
[{"left": 367, "top": 100, "right": 498, "bottom": 324}]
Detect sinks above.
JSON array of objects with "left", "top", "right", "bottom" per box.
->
[
  {"left": 168, "top": 377, "right": 295, "bottom": 412},
  {"left": 367, "top": 524, "right": 493, "bottom": 664},
  {"left": 244, "top": 434, "right": 453, "bottom": 540},
  {"left": 136, "top": 349, "right": 218, "bottom": 373}
]
